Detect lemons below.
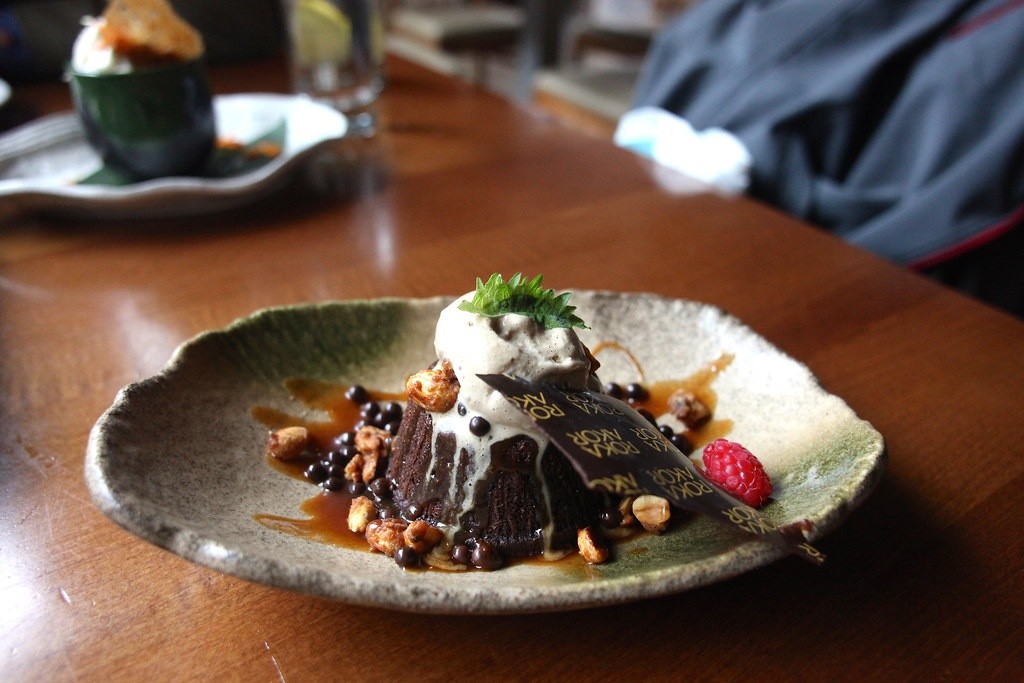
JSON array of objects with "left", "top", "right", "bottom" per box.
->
[{"left": 288, "top": 1, "right": 353, "bottom": 73}]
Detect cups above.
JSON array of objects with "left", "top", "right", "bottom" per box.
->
[
  {"left": 284, "top": 0, "right": 389, "bottom": 134},
  {"left": 59, "top": 47, "right": 216, "bottom": 180}
]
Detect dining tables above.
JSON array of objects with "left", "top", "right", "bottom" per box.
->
[{"left": 0, "top": 52, "right": 1024, "bottom": 683}]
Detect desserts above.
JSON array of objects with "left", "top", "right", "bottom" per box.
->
[
  {"left": 266, "top": 272, "right": 826, "bottom": 576},
  {"left": 63, "top": 0, "right": 210, "bottom": 182}
]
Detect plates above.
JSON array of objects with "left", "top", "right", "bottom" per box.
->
[
  {"left": 81, "top": 289, "right": 887, "bottom": 617},
  {"left": 0, "top": 91, "right": 352, "bottom": 215}
]
